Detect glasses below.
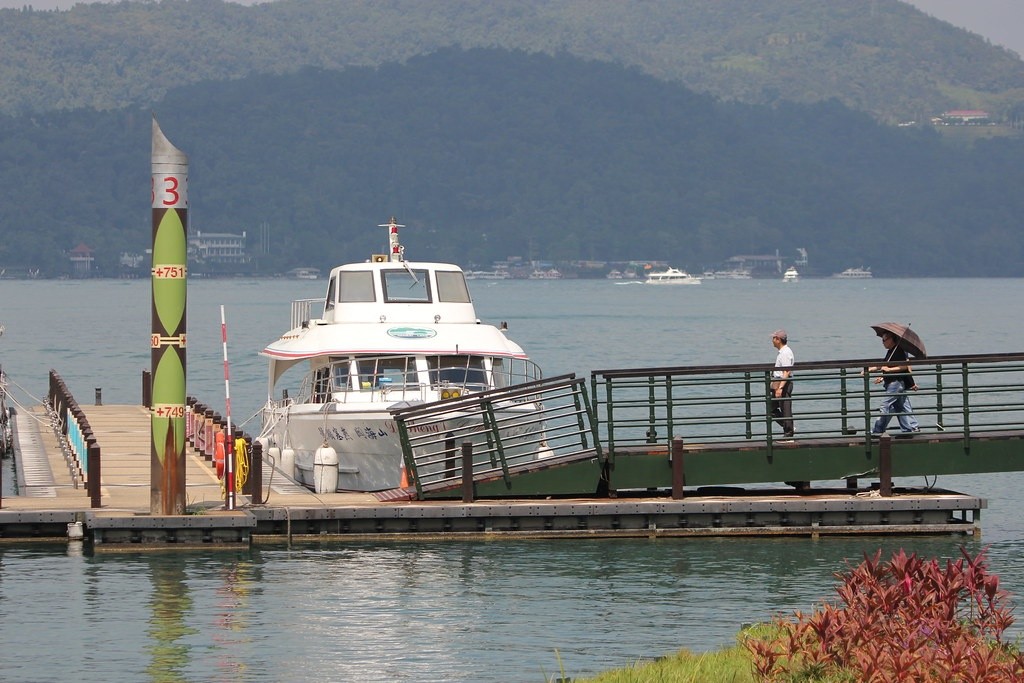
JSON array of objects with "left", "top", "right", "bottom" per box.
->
[{"left": 881, "top": 337, "right": 888, "bottom": 342}]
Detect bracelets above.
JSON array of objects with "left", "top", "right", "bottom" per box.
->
[{"left": 779, "top": 386, "right": 783, "bottom": 388}]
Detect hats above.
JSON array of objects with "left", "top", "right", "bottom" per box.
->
[{"left": 768, "top": 330, "right": 788, "bottom": 340}]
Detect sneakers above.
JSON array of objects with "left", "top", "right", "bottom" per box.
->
[
  {"left": 866, "top": 430, "right": 888, "bottom": 437},
  {"left": 776, "top": 436, "right": 794, "bottom": 443},
  {"left": 899, "top": 430, "right": 920, "bottom": 438}
]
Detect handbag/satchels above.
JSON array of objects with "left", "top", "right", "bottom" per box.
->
[{"left": 769, "top": 378, "right": 782, "bottom": 389}]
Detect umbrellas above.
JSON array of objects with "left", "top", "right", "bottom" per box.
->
[{"left": 870, "top": 321, "right": 927, "bottom": 362}]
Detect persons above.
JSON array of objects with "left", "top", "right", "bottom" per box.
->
[
  {"left": 861, "top": 332, "right": 920, "bottom": 434},
  {"left": 769, "top": 331, "right": 794, "bottom": 443}
]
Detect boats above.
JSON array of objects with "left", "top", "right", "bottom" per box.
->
[
  {"left": 699, "top": 271, "right": 714, "bottom": 278},
  {"left": 715, "top": 270, "right": 752, "bottom": 279},
  {"left": 609, "top": 268, "right": 623, "bottom": 279},
  {"left": 782, "top": 265, "right": 800, "bottom": 282},
  {"left": 297, "top": 270, "right": 318, "bottom": 280},
  {"left": 645, "top": 266, "right": 702, "bottom": 284},
  {"left": 528, "top": 269, "right": 546, "bottom": 279},
  {"left": 833, "top": 263, "right": 872, "bottom": 278},
  {"left": 543, "top": 267, "right": 561, "bottom": 280},
  {"left": 260, "top": 216, "right": 554, "bottom": 494},
  {"left": 464, "top": 269, "right": 511, "bottom": 279}
]
